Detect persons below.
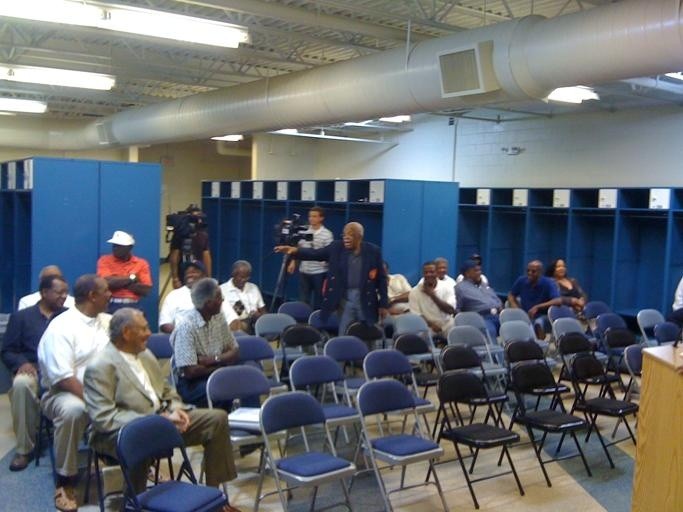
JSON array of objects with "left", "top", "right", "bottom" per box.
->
[
  {"left": 0, "top": 273, "right": 71, "bottom": 472},
  {"left": 371, "top": 251, "right": 590, "bottom": 377},
  {"left": 670, "top": 275, "right": 682, "bottom": 329},
  {"left": 157, "top": 259, "right": 241, "bottom": 335},
  {"left": 168, "top": 206, "right": 213, "bottom": 290},
  {"left": 37, "top": 269, "right": 168, "bottom": 512},
  {"left": 82, "top": 310, "right": 239, "bottom": 512},
  {"left": 286, "top": 206, "right": 337, "bottom": 314},
  {"left": 17, "top": 264, "right": 77, "bottom": 312},
  {"left": 272, "top": 222, "right": 392, "bottom": 373},
  {"left": 96, "top": 229, "right": 153, "bottom": 317},
  {"left": 218, "top": 260, "right": 269, "bottom": 336},
  {"left": 169, "top": 278, "right": 266, "bottom": 459}
]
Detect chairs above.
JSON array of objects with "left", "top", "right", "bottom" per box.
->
[
  {"left": 170, "top": 346, "right": 196, "bottom": 404},
  {"left": 322, "top": 335, "right": 379, "bottom": 445},
  {"left": 277, "top": 300, "right": 315, "bottom": 325},
  {"left": 117, "top": 416, "right": 229, "bottom": 512},
  {"left": 255, "top": 311, "right": 305, "bottom": 384},
  {"left": 306, "top": 307, "right": 344, "bottom": 371},
  {"left": 36, "top": 375, "right": 93, "bottom": 486},
  {"left": 255, "top": 391, "right": 358, "bottom": 512},
  {"left": 288, "top": 355, "right": 357, "bottom": 461},
  {"left": 347, "top": 287, "right": 683, "bottom": 508},
  {"left": 207, "top": 365, "right": 287, "bottom": 509},
  {"left": 81, "top": 400, "right": 173, "bottom": 512},
  {"left": 280, "top": 324, "right": 328, "bottom": 389},
  {"left": 230, "top": 327, "right": 268, "bottom": 374},
  {"left": 145, "top": 332, "right": 184, "bottom": 404},
  {"left": 234, "top": 334, "right": 288, "bottom": 396}
]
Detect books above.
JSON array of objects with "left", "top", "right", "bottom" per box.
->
[{"left": 225, "top": 407, "right": 262, "bottom": 433}]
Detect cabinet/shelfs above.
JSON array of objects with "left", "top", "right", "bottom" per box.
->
[
  {"left": 0, "top": 154, "right": 161, "bottom": 333},
  {"left": 459, "top": 185, "right": 682, "bottom": 337},
  {"left": 199, "top": 178, "right": 459, "bottom": 309}
]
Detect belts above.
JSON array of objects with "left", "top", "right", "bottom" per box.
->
[{"left": 110, "top": 297, "right": 137, "bottom": 303}]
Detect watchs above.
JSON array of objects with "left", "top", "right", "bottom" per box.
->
[
  {"left": 214, "top": 351, "right": 222, "bottom": 365},
  {"left": 128, "top": 272, "right": 137, "bottom": 284}
]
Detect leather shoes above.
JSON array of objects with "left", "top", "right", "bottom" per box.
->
[
  {"left": 10, "top": 453, "right": 30, "bottom": 471},
  {"left": 54, "top": 486, "right": 77, "bottom": 511}
]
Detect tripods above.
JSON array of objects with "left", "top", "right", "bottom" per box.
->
[{"left": 268, "top": 250, "right": 313, "bottom": 310}]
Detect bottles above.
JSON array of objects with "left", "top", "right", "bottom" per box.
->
[{"left": 211, "top": 347, "right": 221, "bottom": 364}]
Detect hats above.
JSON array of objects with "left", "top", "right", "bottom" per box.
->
[
  {"left": 180, "top": 260, "right": 206, "bottom": 273},
  {"left": 106, "top": 230, "right": 135, "bottom": 246},
  {"left": 461, "top": 260, "right": 475, "bottom": 272}
]
